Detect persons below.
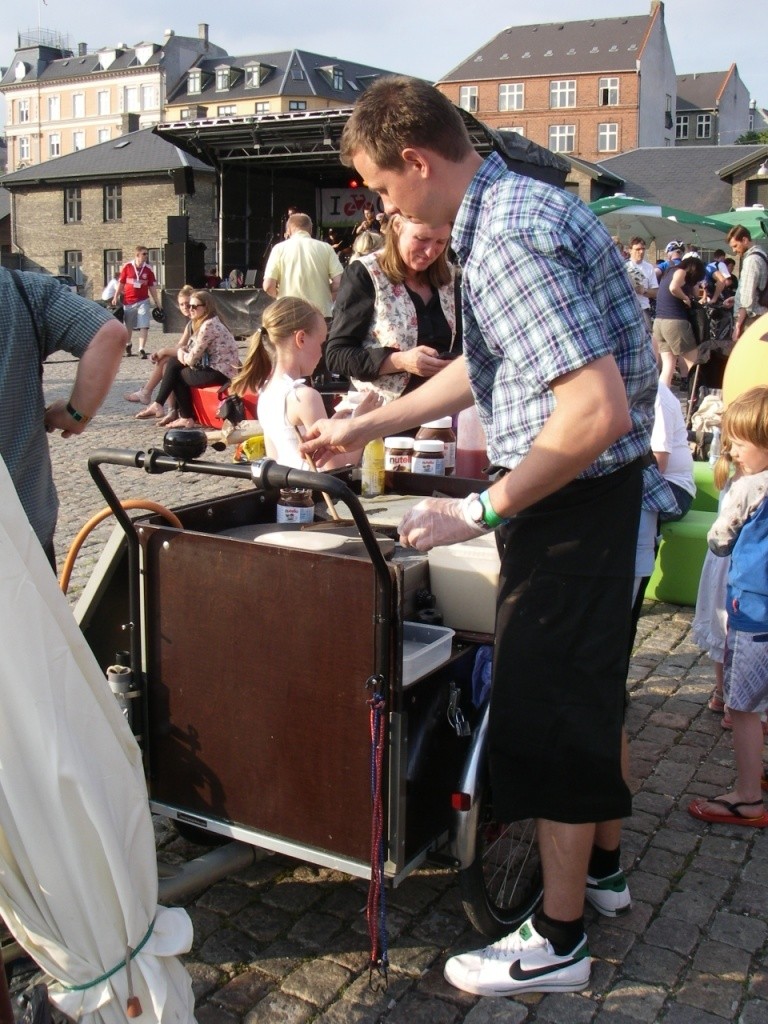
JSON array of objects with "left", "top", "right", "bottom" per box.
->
[
  {"left": 124, "top": 285, "right": 240, "bottom": 430},
  {"left": 287, "top": 204, "right": 383, "bottom": 269},
  {"left": 609, "top": 225, "right": 768, "bottom": 393},
  {"left": 101, "top": 245, "right": 164, "bottom": 360},
  {"left": 295, "top": 80, "right": 659, "bottom": 1000},
  {"left": 630, "top": 352, "right": 768, "bottom": 827},
  {"left": 263, "top": 213, "right": 344, "bottom": 418},
  {"left": 324, "top": 213, "right": 463, "bottom": 437},
  {"left": 0, "top": 264, "right": 131, "bottom": 577},
  {"left": 230, "top": 296, "right": 385, "bottom": 472}
]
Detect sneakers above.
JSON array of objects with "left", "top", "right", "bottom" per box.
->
[
  {"left": 125, "top": 344, "right": 132, "bottom": 356},
  {"left": 585, "top": 869, "right": 634, "bottom": 917},
  {"left": 139, "top": 350, "right": 146, "bottom": 358},
  {"left": 443, "top": 914, "right": 591, "bottom": 996}
]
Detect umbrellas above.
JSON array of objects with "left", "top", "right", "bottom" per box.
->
[
  {"left": 586, "top": 194, "right": 738, "bottom": 257},
  {"left": 704, "top": 210, "right": 768, "bottom": 240}
]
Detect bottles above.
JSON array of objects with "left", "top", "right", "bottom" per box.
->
[
  {"left": 455, "top": 404, "right": 491, "bottom": 480},
  {"left": 361, "top": 436, "right": 385, "bottom": 497}
]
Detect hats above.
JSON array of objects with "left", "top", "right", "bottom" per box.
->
[{"left": 682, "top": 251, "right": 703, "bottom": 260}]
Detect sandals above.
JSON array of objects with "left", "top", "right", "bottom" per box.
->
[
  {"left": 135, "top": 410, "right": 161, "bottom": 419},
  {"left": 689, "top": 795, "right": 768, "bottom": 828},
  {"left": 156, "top": 413, "right": 179, "bottom": 426},
  {"left": 123, "top": 387, "right": 152, "bottom": 404},
  {"left": 165, "top": 419, "right": 194, "bottom": 428}
]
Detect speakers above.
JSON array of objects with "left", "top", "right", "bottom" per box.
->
[
  {"left": 173, "top": 167, "right": 196, "bottom": 195},
  {"left": 164, "top": 242, "right": 205, "bottom": 289},
  {"left": 243, "top": 270, "right": 264, "bottom": 288},
  {"left": 167, "top": 216, "right": 189, "bottom": 243}
]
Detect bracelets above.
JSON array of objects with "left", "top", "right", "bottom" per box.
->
[
  {"left": 67, "top": 402, "right": 93, "bottom": 424},
  {"left": 479, "top": 489, "right": 503, "bottom": 528}
]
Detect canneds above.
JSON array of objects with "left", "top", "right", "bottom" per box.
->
[
  {"left": 384, "top": 415, "right": 457, "bottom": 477},
  {"left": 277, "top": 487, "right": 315, "bottom": 523}
]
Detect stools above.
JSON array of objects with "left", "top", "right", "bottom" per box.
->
[{"left": 641, "top": 460, "right": 737, "bottom": 603}]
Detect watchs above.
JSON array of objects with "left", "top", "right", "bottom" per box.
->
[{"left": 467, "top": 495, "right": 491, "bottom": 530}]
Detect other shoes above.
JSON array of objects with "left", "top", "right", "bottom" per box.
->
[
  {"left": 707, "top": 689, "right": 725, "bottom": 712},
  {"left": 720, "top": 717, "right": 768, "bottom": 733}
]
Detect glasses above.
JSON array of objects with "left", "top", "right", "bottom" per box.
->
[
  {"left": 139, "top": 252, "right": 149, "bottom": 256},
  {"left": 186, "top": 304, "right": 206, "bottom": 310}
]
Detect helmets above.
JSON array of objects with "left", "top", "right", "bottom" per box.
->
[{"left": 664, "top": 239, "right": 686, "bottom": 253}]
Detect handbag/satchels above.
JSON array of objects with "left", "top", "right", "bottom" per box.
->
[
  {"left": 217, "top": 393, "right": 245, "bottom": 424},
  {"left": 187, "top": 351, "right": 209, "bottom": 370}
]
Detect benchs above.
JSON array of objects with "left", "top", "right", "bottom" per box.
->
[{"left": 190, "top": 381, "right": 260, "bottom": 429}]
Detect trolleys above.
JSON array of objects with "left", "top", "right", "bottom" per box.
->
[{"left": 74, "top": 428, "right": 543, "bottom": 943}]
